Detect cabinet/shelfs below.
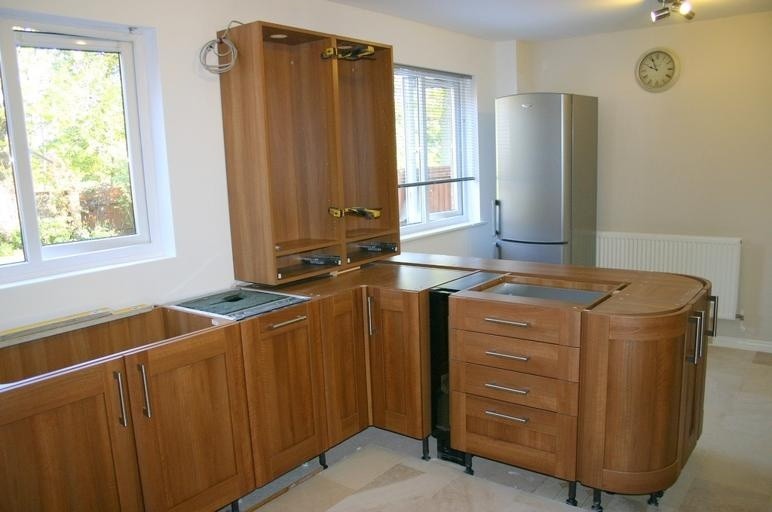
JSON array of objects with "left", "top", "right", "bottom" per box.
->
[
  {"left": 215, "top": 22, "right": 399, "bottom": 286},
  {"left": 0, "top": 320, "right": 255, "bottom": 508},
  {"left": 240, "top": 287, "right": 428, "bottom": 494},
  {"left": 450, "top": 283, "right": 716, "bottom": 512}
]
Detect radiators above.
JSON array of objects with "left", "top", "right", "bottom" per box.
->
[{"left": 594, "top": 231, "right": 744, "bottom": 320}]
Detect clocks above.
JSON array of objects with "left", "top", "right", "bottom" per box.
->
[{"left": 634, "top": 47, "right": 678, "bottom": 93}]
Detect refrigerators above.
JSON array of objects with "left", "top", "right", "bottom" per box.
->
[{"left": 492, "top": 91, "right": 599, "bottom": 265}]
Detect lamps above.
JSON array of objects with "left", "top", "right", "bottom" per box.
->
[{"left": 651, "top": 0, "right": 695, "bottom": 22}]
